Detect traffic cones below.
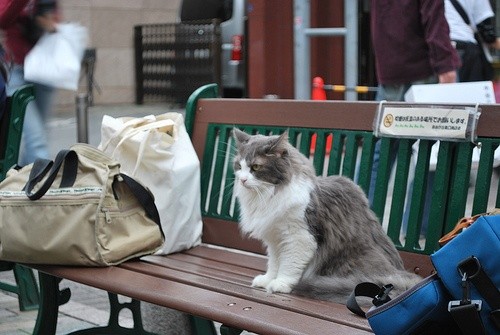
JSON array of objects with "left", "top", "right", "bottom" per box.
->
[{"left": 302, "top": 76, "right": 346, "bottom": 157}]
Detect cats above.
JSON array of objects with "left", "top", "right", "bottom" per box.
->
[{"left": 230, "top": 124, "right": 426, "bottom": 301}]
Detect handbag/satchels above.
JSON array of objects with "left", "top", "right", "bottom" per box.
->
[
  {"left": 23, "top": 23, "right": 87, "bottom": 92},
  {"left": 0, "top": 144, "right": 165, "bottom": 267},
  {"left": 346, "top": 211, "right": 500, "bottom": 335},
  {"left": 97, "top": 112, "right": 203, "bottom": 256}
]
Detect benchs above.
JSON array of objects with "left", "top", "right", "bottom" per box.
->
[{"left": 15, "top": 83, "right": 500, "bottom": 335}]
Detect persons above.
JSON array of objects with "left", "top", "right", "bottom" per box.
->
[
  {"left": 358, "top": 0, "right": 500, "bottom": 243},
  {"left": 0, "top": 0, "right": 61, "bottom": 166}
]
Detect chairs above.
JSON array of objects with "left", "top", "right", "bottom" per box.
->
[{"left": 0, "top": 84, "right": 53, "bottom": 313}]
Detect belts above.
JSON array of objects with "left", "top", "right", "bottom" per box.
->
[{"left": 451, "top": 40, "right": 474, "bottom": 49}]
